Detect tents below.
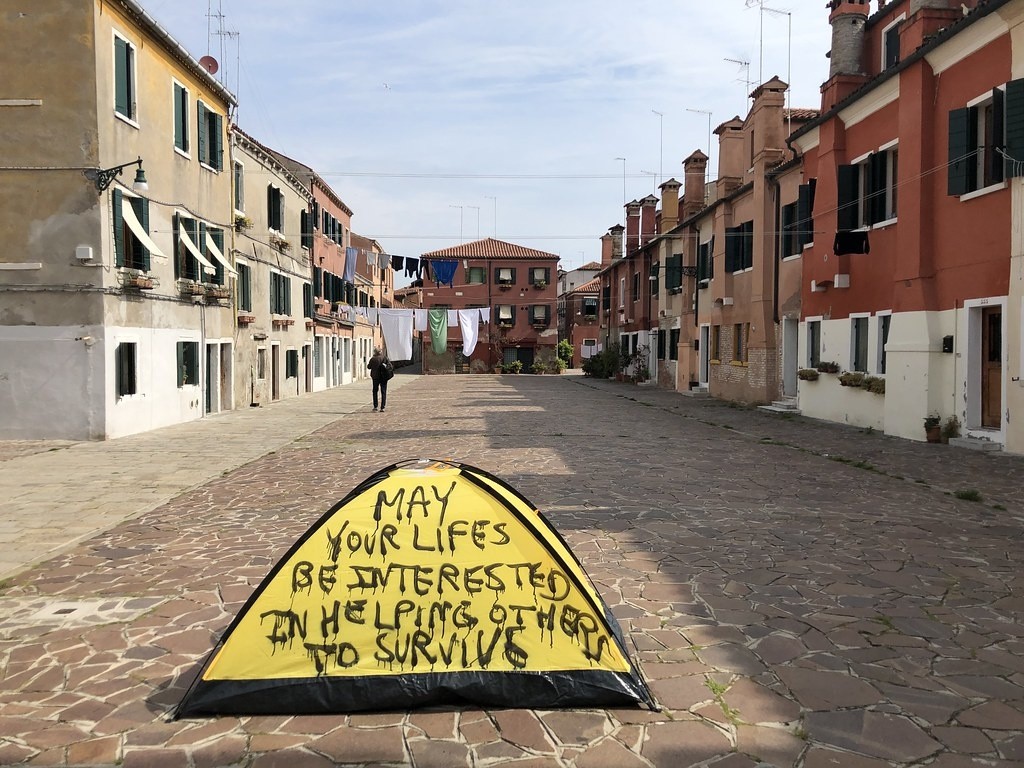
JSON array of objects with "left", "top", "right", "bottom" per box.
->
[{"left": 163, "top": 457, "right": 664, "bottom": 727}]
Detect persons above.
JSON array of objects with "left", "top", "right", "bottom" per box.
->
[{"left": 366, "top": 347, "right": 394, "bottom": 412}]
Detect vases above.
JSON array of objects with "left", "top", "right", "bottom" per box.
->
[{"left": 495, "top": 367, "right": 502, "bottom": 373}]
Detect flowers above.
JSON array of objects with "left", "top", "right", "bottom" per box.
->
[{"left": 487, "top": 322, "right": 522, "bottom": 367}]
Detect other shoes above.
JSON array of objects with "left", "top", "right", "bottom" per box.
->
[
  {"left": 372, "top": 409, "right": 378, "bottom": 413},
  {"left": 380, "top": 409, "right": 385, "bottom": 412}
]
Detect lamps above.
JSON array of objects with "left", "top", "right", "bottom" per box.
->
[
  {"left": 382, "top": 283, "right": 388, "bottom": 295},
  {"left": 344, "top": 282, "right": 363, "bottom": 292},
  {"left": 649, "top": 266, "right": 701, "bottom": 282},
  {"left": 97, "top": 156, "right": 149, "bottom": 196}
]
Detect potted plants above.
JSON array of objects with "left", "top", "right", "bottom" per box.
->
[
  {"left": 536, "top": 281, "right": 546, "bottom": 290},
  {"left": 922, "top": 412, "right": 943, "bottom": 443},
  {"left": 539, "top": 362, "right": 548, "bottom": 374},
  {"left": 796, "top": 361, "right": 885, "bottom": 395},
  {"left": 580, "top": 342, "right": 651, "bottom": 385},
  {"left": 511, "top": 360, "right": 523, "bottom": 374},
  {"left": 235, "top": 215, "right": 255, "bottom": 234},
  {"left": 554, "top": 357, "right": 568, "bottom": 374},
  {"left": 503, "top": 364, "right": 513, "bottom": 373},
  {"left": 531, "top": 363, "right": 539, "bottom": 375},
  {"left": 280, "top": 240, "right": 293, "bottom": 254},
  {"left": 941, "top": 414, "right": 961, "bottom": 444}
]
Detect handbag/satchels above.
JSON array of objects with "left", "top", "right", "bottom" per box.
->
[{"left": 380, "top": 356, "right": 393, "bottom": 380}]
